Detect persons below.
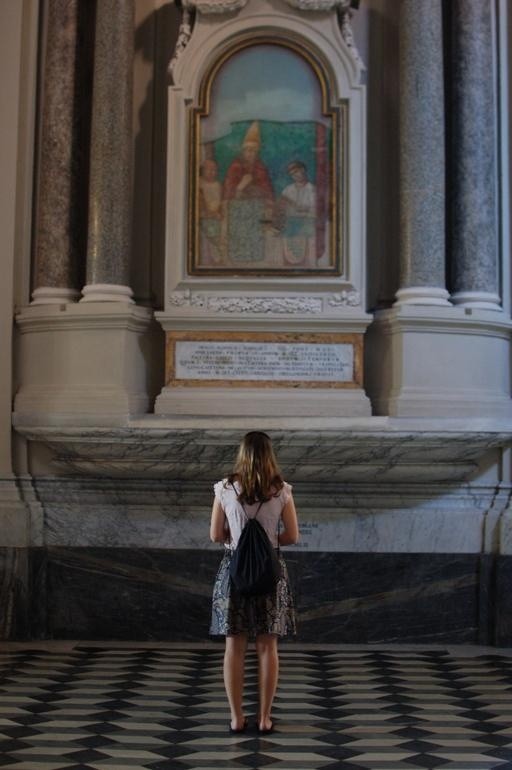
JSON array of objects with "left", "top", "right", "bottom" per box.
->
[
  {"left": 223, "top": 146, "right": 275, "bottom": 269},
  {"left": 208, "top": 430, "right": 300, "bottom": 733},
  {"left": 197, "top": 160, "right": 225, "bottom": 266},
  {"left": 276, "top": 160, "right": 318, "bottom": 269}
]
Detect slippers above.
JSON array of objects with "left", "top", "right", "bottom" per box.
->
[
  {"left": 254, "top": 717, "right": 275, "bottom": 735},
  {"left": 227, "top": 716, "right": 250, "bottom": 736}
]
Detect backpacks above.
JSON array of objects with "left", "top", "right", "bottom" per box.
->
[{"left": 224, "top": 478, "right": 285, "bottom": 600}]
